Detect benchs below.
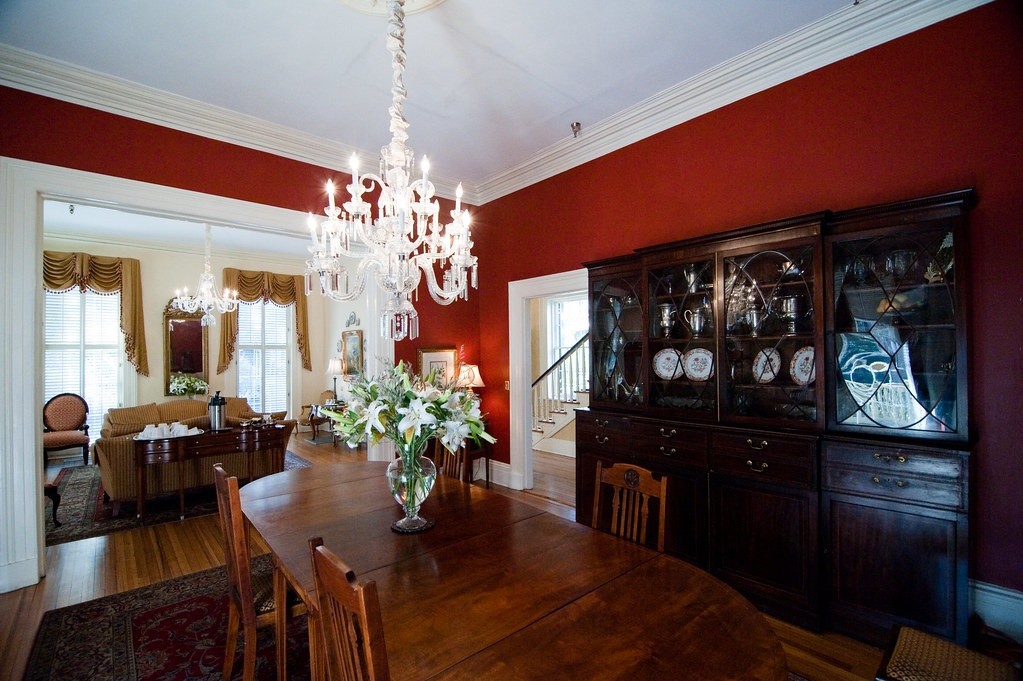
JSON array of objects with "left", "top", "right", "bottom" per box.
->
[{"left": 211, "top": 463, "right": 305, "bottom": 681}]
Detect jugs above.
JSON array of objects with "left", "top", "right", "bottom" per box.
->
[
  {"left": 684, "top": 263, "right": 699, "bottom": 293},
  {"left": 208, "top": 391, "right": 227, "bottom": 432},
  {"left": 684, "top": 307, "right": 711, "bottom": 340},
  {"left": 776, "top": 257, "right": 798, "bottom": 275},
  {"left": 624, "top": 295, "right": 636, "bottom": 306}
]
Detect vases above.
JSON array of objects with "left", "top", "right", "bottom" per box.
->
[{"left": 385, "top": 441, "right": 436, "bottom": 535}]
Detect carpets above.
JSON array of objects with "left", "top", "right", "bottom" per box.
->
[
  {"left": 45, "top": 431, "right": 315, "bottom": 547},
  {"left": 19, "top": 552, "right": 311, "bottom": 680}
]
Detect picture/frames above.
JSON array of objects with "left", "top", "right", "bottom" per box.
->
[
  {"left": 416, "top": 348, "right": 458, "bottom": 395},
  {"left": 342, "top": 330, "right": 363, "bottom": 382}
]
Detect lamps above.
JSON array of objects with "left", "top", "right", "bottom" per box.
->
[
  {"left": 325, "top": 358, "right": 343, "bottom": 399},
  {"left": 176, "top": 224, "right": 240, "bottom": 326},
  {"left": 306, "top": 0, "right": 478, "bottom": 341},
  {"left": 456, "top": 363, "right": 485, "bottom": 420}
]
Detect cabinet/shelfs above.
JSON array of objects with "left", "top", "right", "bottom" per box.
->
[
  {"left": 630, "top": 240, "right": 818, "bottom": 634},
  {"left": 573, "top": 252, "right": 629, "bottom": 539},
  {"left": 822, "top": 187, "right": 987, "bottom": 655}
]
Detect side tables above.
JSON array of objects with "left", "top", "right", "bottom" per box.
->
[{"left": 309, "top": 404, "right": 348, "bottom": 447}]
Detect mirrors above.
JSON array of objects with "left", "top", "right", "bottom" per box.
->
[{"left": 164, "top": 295, "right": 208, "bottom": 394}]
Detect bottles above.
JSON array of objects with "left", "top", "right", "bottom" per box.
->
[
  {"left": 665, "top": 279, "right": 673, "bottom": 295},
  {"left": 326, "top": 399, "right": 335, "bottom": 405}
]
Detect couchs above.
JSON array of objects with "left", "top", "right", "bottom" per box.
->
[{"left": 91, "top": 397, "right": 296, "bottom": 516}]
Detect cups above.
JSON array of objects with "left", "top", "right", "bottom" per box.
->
[
  {"left": 251, "top": 418, "right": 263, "bottom": 427},
  {"left": 144, "top": 422, "right": 188, "bottom": 439},
  {"left": 240, "top": 419, "right": 251, "bottom": 427}
]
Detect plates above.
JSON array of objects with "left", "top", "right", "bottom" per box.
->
[
  {"left": 683, "top": 348, "right": 714, "bottom": 381},
  {"left": 790, "top": 346, "right": 816, "bottom": 385},
  {"left": 752, "top": 348, "right": 781, "bottom": 383},
  {"left": 652, "top": 348, "right": 685, "bottom": 380}
]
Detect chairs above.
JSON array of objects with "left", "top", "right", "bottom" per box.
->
[
  {"left": 435, "top": 437, "right": 472, "bottom": 483},
  {"left": 588, "top": 460, "right": 670, "bottom": 554},
  {"left": 307, "top": 536, "right": 390, "bottom": 680},
  {"left": 43, "top": 393, "right": 90, "bottom": 527},
  {"left": 295, "top": 390, "right": 337, "bottom": 441}
]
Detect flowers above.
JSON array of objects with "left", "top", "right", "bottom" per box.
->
[
  {"left": 317, "top": 355, "right": 497, "bottom": 441},
  {"left": 169, "top": 371, "right": 210, "bottom": 395}
]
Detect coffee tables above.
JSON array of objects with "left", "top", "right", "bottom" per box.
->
[{"left": 134, "top": 420, "right": 285, "bottom": 520}]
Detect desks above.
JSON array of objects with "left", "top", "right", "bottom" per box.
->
[{"left": 239, "top": 461, "right": 786, "bottom": 680}]
